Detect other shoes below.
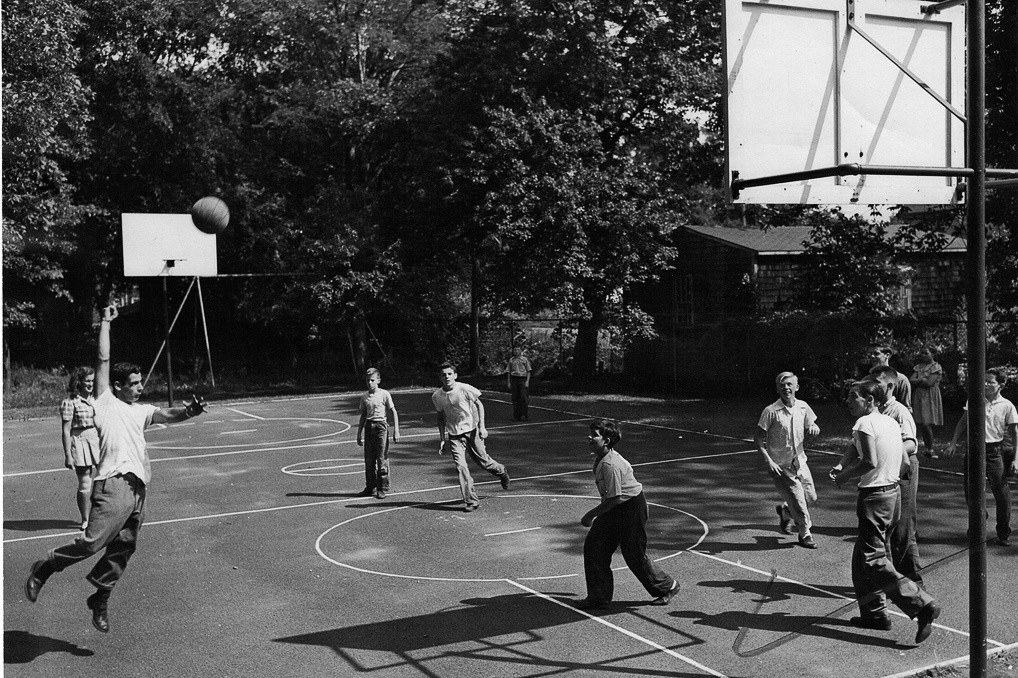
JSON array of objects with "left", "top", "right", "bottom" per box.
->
[
  {"left": 997, "top": 533, "right": 1012, "bottom": 546},
  {"left": 463, "top": 503, "right": 479, "bottom": 512},
  {"left": 358, "top": 486, "right": 376, "bottom": 497},
  {"left": 499, "top": 466, "right": 510, "bottom": 489},
  {"left": 925, "top": 451, "right": 939, "bottom": 460},
  {"left": 525, "top": 416, "right": 531, "bottom": 421},
  {"left": 798, "top": 534, "right": 818, "bottom": 549},
  {"left": 512, "top": 417, "right": 521, "bottom": 422},
  {"left": 915, "top": 601, "right": 941, "bottom": 644},
  {"left": 850, "top": 617, "right": 893, "bottom": 631},
  {"left": 574, "top": 599, "right": 611, "bottom": 611},
  {"left": 651, "top": 580, "right": 680, "bottom": 606},
  {"left": 967, "top": 510, "right": 988, "bottom": 537},
  {"left": 376, "top": 490, "right": 386, "bottom": 499},
  {"left": 81, "top": 521, "right": 88, "bottom": 530},
  {"left": 776, "top": 505, "right": 793, "bottom": 535}
]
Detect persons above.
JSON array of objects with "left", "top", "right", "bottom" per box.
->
[
  {"left": 509, "top": 346, "right": 531, "bottom": 422},
  {"left": 575, "top": 416, "right": 682, "bottom": 612},
  {"left": 829, "top": 343, "right": 1018, "bottom": 644},
  {"left": 26, "top": 297, "right": 208, "bottom": 633},
  {"left": 61, "top": 366, "right": 99, "bottom": 530},
  {"left": 754, "top": 372, "right": 820, "bottom": 550},
  {"left": 432, "top": 362, "right": 509, "bottom": 512},
  {"left": 358, "top": 368, "right": 400, "bottom": 499}
]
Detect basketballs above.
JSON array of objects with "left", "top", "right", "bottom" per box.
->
[{"left": 191, "top": 197, "right": 230, "bottom": 234}]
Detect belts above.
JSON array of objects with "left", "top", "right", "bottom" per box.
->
[{"left": 858, "top": 482, "right": 898, "bottom": 493}]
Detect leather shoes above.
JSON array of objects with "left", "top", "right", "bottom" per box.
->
[
  {"left": 87, "top": 592, "right": 109, "bottom": 632},
  {"left": 24, "top": 560, "right": 46, "bottom": 602}
]
